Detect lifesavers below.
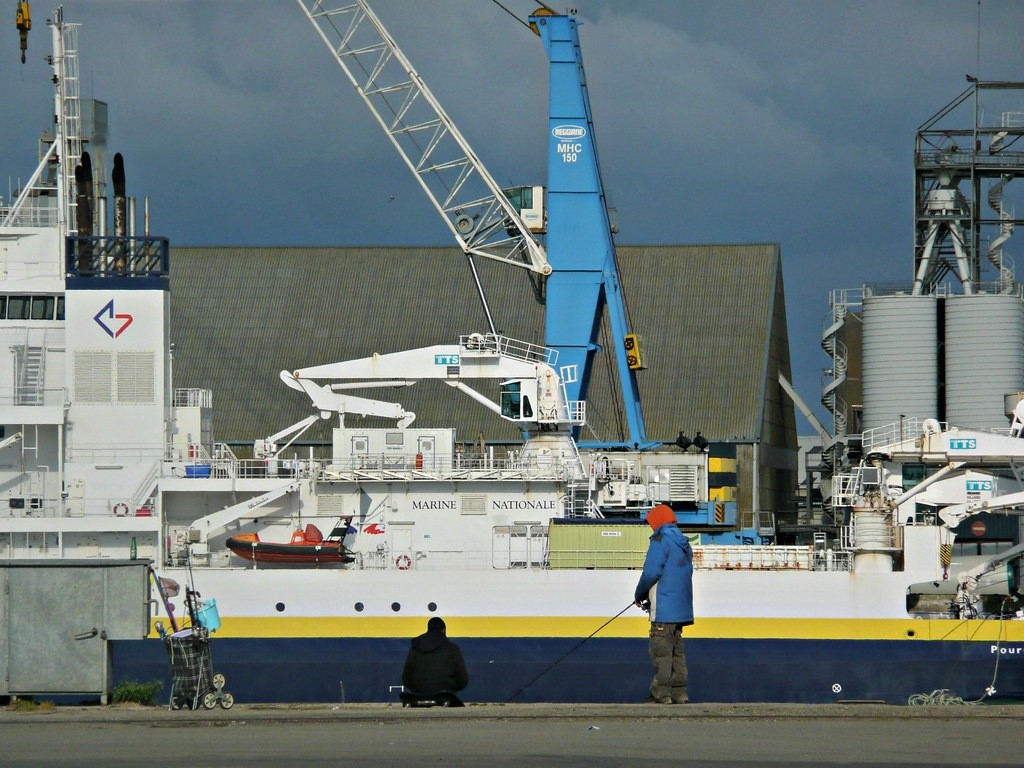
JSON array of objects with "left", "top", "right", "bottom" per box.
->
[
  {"left": 396, "top": 554, "right": 411, "bottom": 570},
  {"left": 113, "top": 503, "right": 129, "bottom": 517}
]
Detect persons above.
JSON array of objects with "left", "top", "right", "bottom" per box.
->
[
  {"left": 955, "top": 581, "right": 979, "bottom": 619},
  {"left": 634, "top": 505, "right": 694, "bottom": 706},
  {"left": 922, "top": 508, "right": 934, "bottom": 526},
  {"left": 400, "top": 617, "right": 468, "bottom": 709}
]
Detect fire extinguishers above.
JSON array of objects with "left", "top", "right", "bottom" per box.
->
[{"left": 416, "top": 452, "right": 423, "bottom": 469}]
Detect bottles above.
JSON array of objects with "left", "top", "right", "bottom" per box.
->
[{"left": 130, "top": 536, "right": 137, "bottom": 560}]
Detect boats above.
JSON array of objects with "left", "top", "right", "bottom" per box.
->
[{"left": 224, "top": 517, "right": 354, "bottom": 564}]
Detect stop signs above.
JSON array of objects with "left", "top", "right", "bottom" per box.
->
[{"left": 973, "top": 521, "right": 985, "bottom": 538}]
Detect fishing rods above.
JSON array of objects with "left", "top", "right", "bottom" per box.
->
[
  {"left": 182, "top": 531, "right": 201, "bottom": 628},
  {"left": 505, "top": 596, "right": 646, "bottom": 703}
]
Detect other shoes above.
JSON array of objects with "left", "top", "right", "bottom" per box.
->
[
  {"left": 671, "top": 695, "right": 688, "bottom": 703},
  {"left": 639, "top": 695, "right": 672, "bottom": 704}
]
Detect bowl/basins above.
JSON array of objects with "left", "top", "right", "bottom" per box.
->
[{"left": 184, "top": 465, "right": 211, "bottom": 478}]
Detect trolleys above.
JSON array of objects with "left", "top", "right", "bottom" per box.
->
[{"left": 146, "top": 565, "right": 234, "bottom": 711}]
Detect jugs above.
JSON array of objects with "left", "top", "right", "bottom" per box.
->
[{"left": 335, "top": 679, "right": 346, "bottom": 703}]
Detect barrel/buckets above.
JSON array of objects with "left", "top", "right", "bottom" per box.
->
[{"left": 184, "top": 599, "right": 222, "bottom": 633}]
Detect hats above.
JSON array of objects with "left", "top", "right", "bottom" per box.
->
[{"left": 647, "top": 505, "right": 677, "bottom": 531}]
